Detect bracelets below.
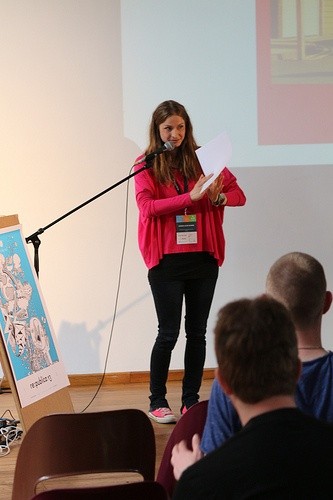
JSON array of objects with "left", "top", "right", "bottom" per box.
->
[{"left": 210, "top": 194, "right": 225, "bottom": 205}]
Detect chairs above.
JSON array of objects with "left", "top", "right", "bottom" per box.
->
[
  {"left": 30, "top": 481, "right": 169, "bottom": 500},
  {"left": 12, "top": 409, "right": 155, "bottom": 500},
  {"left": 156, "top": 400, "right": 209, "bottom": 500}
]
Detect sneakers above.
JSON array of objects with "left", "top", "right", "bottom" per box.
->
[
  {"left": 181, "top": 405, "right": 195, "bottom": 415},
  {"left": 148, "top": 407, "right": 178, "bottom": 424}
]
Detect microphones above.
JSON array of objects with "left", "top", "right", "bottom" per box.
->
[{"left": 133, "top": 141, "right": 176, "bottom": 165}]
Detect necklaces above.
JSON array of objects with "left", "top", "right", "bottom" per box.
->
[{"left": 297, "top": 345, "right": 322, "bottom": 349}]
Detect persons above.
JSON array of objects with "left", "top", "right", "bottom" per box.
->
[
  {"left": 134, "top": 100, "right": 246, "bottom": 424},
  {"left": 170, "top": 294, "right": 333, "bottom": 500},
  {"left": 200, "top": 252, "right": 333, "bottom": 455}
]
